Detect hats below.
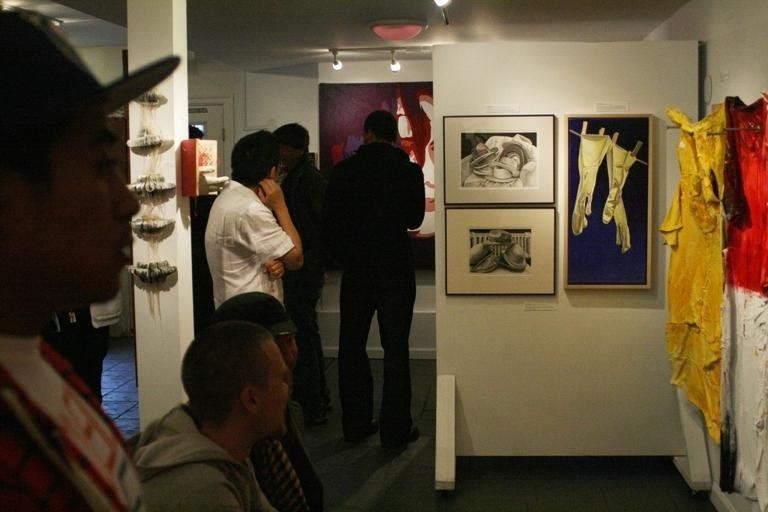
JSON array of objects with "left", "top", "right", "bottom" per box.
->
[
  {"left": 470, "top": 229, "right": 526, "bottom": 272},
  {"left": 270, "top": 124, "right": 309, "bottom": 150},
  {"left": 470, "top": 134, "right": 532, "bottom": 183},
  {"left": 213, "top": 292, "right": 299, "bottom": 341},
  {"left": 0, "top": 10, "right": 180, "bottom": 154}
]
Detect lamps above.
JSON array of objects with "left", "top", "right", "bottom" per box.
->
[
  {"left": 328, "top": 45, "right": 402, "bottom": 72},
  {"left": 369, "top": 18, "right": 429, "bottom": 43}
]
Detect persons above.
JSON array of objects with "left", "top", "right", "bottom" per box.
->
[
  {"left": 266, "top": 119, "right": 334, "bottom": 428},
  {"left": 39, "top": 283, "right": 122, "bottom": 406},
  {"left": 189, "top": 124, "right": 221, "bottom": 336},
  {"left": 206, "top": 288, "right": 327, "bottom": 512},
  {"left": 0, "top": 5, "right": 182, "bottom": 511},
  {"left": 200, "top": 128, "right": 304, "bottom": 315},
  {"left": 318, "top": 108, "right": 427, "bottom": 451},
  {"left": 119, "top": 317, "right": 292, "bottom": 512}
]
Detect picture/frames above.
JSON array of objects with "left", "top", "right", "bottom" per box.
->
[
  {"left": 443, "top": 114, "right": 556, "bottom": 206},
  {"left": 445, "top": 207, "right": 555, "bottom": 296},
  {"left": 563, "top": 114, "right": 652, "bottom": 289}
]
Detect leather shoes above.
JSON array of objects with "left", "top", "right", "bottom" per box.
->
[
  {"left": 343, "top": 419, "right": 377, "bottom": 445},
  {"left": 407, "top": 426, "right": 419, "bottom": 441}
]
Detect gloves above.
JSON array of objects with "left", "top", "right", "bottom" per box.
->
[
  {"left": 572, "top": 134, "right": 611, "bottom": 235},
  {"left": 604, "top": 143, "right": 636, "bottom": 253}
]
